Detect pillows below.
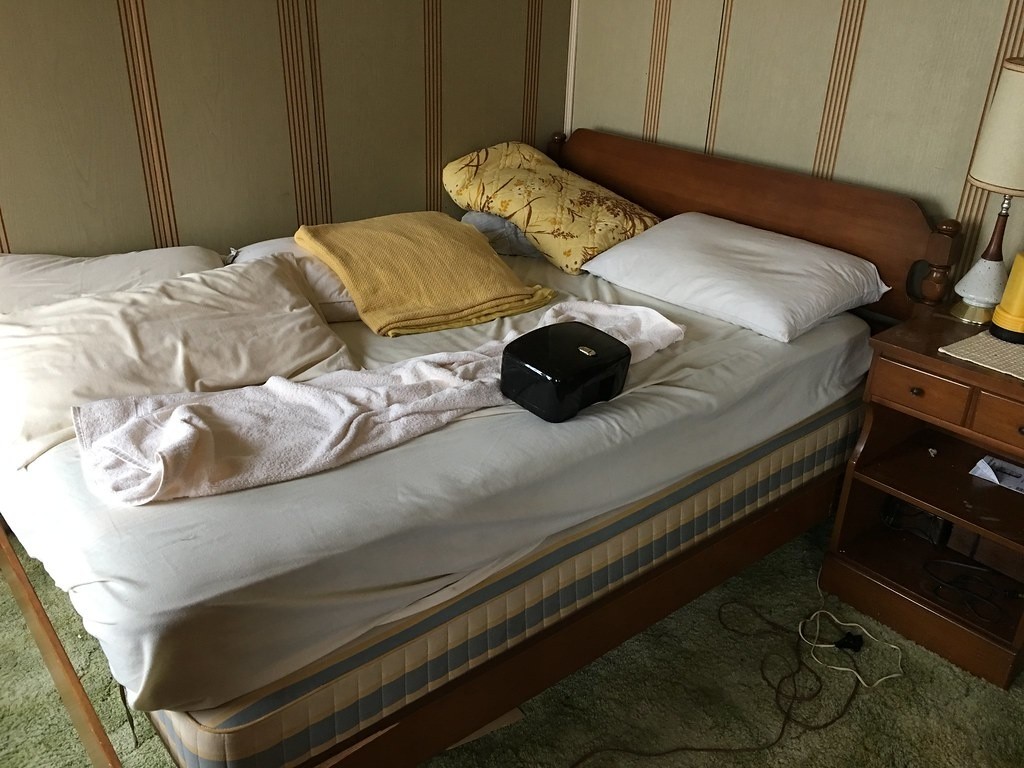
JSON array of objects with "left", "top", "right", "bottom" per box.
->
[
  {"left": 0, "top": 250, "right": 363, "bottom": 471},
  {"left": 231, "top": 239, "right": 361, "bottom": 322},
  {"left": 0, "top": 246, "right": 225, "bottom": 315},
  {"left": 580, "top": 211, "right": 892, "bottom": 343}
]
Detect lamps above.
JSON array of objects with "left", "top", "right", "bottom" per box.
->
[{"left": 951, "top": 58, "right": 1024, "bottom": 324}]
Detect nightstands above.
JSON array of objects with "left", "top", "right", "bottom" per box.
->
[{"left": 819, "top": 312, "right": 1024, "bottom": 691}]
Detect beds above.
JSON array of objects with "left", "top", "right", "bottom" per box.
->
[{"left": 0, "top": 127, "right": 966, "bottom": 768}]
